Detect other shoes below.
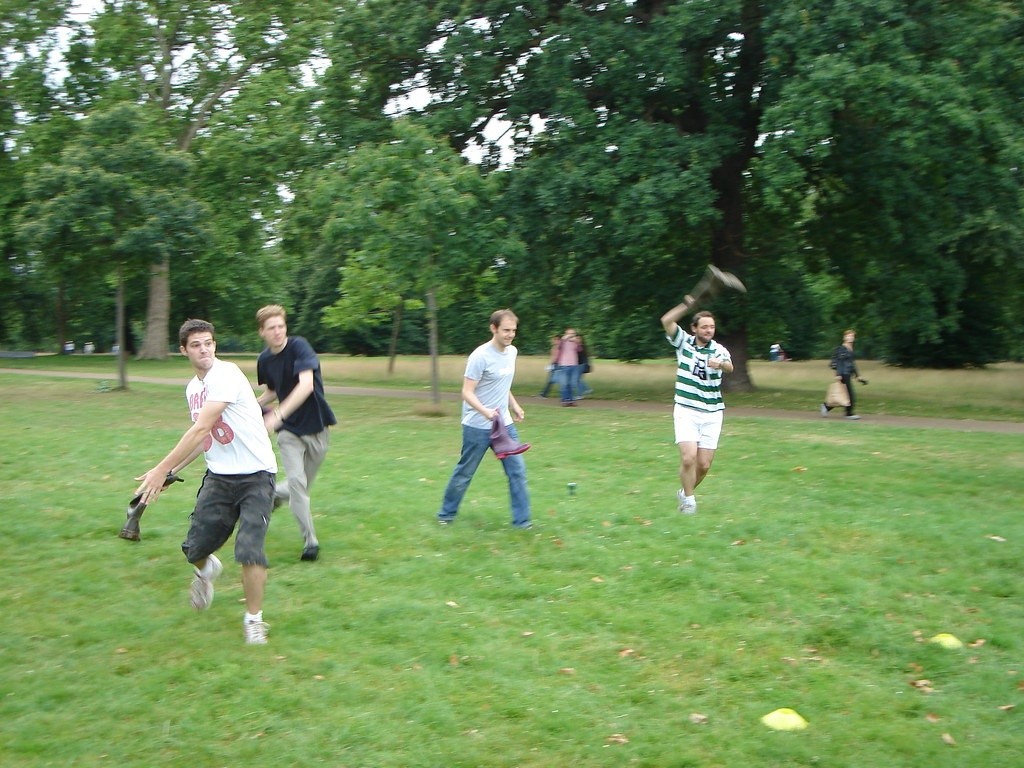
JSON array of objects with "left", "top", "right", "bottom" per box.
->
[
  {"left": 820, "top": 404, "right": 828, "bottom": 418},
  {"left": 301, "top": 546, "right": 315, "bottom": 561},
  {"left": 845, "top": 415, "right": 860, "bottom": 420}
]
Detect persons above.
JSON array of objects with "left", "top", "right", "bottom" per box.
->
[
  {"left": 252, "top": 305, "right": 336, "bottom": 562},
  {"left": 660, "top": 296, "right": 735, "bottom": 513},
  {"left": 132, "top": 319, "right": 277, "bottom": 646},
  {"left": 539, "top": 328, "right": 594, "bottom": 406},
  {"left": 821, "top": 329, "right": 870, "bottom": 420},
  {"left": 436, "top": 307, "right": 533, "bottom": 531}
]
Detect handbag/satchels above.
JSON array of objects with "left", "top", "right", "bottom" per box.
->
[
  {"left": 826, "top": 381, "right": 851, "bottom": 407},
  {"left": 583, "top": 365, "right": 590, "bottom": 373}
]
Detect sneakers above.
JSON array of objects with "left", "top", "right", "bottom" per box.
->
[
  {"left": 677, "top": 487, "right": 696, "bottom": 514},
  {"left": 244, "top": 620, "right": 271, "bottom": 645},
  {"left": 190, "top": 554, "right": 223, "bottom": 612}
]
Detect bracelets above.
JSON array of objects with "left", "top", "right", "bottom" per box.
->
[{"left": 682, "top": 299, "right": 690, "bottom": 306}]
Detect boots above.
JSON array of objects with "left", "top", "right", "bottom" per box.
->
[
  {"left": 687, "top": 263, "right": 747, "bottom": 303},
  {"left": 120, "top": 474, "right": 184, "bottom": 542},
  {"left": 490, "top": 409, "right": 531, "bottom": 459}
]
